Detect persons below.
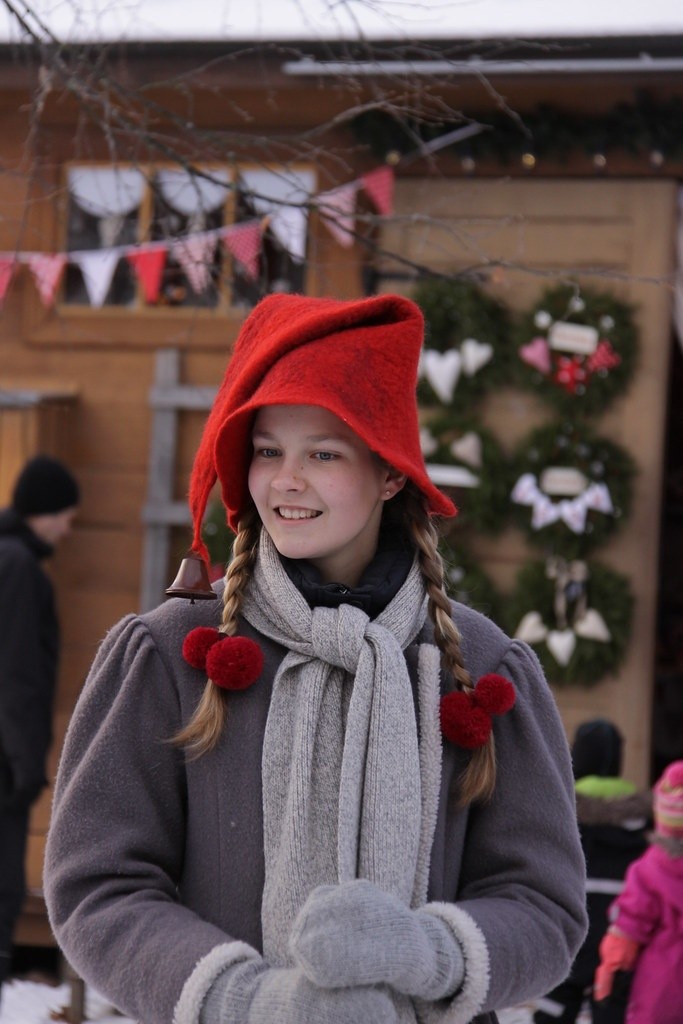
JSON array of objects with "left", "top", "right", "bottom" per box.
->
[
  {"left": 529, "top": 717, "right": 655, "bottom": 1024},
  {"left": 593, "top": 761, "right": 682, "bottom": 1024},
  {"left": 0, "top": 454, "right": 84, "bottom": 983},
  {"left": 44, "top": 291, "right": 590, "bottom": 1024}
]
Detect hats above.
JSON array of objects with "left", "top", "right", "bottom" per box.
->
[
  {"left": 10, "top": 454, "right": 84, "bottom": 520},
  {"left": 163, "top": 292, "right": 458, "bottom": 560},
  {"left": 571, "top": 720, "right": 622, "bottom": 781},
  {"left": 651, "top": 760, "right": 683, "bottom": 841}
]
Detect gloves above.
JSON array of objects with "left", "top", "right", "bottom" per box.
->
[
  {"left": 290, "top": 876, "right": 465, "bottom": 1002},
  {"left": 198, "top": 958, "right": 419, "bottom": 1024},
  {"left": 594, "top": 933, "right": 636, "bottom": 1001}
]
